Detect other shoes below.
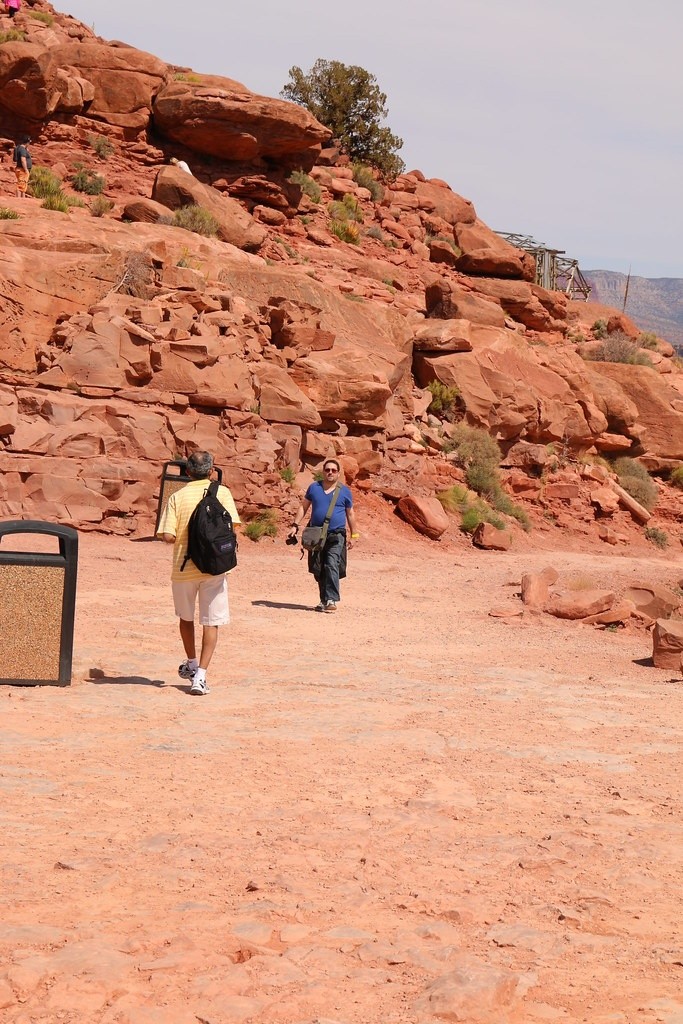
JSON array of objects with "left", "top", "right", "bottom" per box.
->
[
  {"left": 324, "top": 600, "right": 337, "bottom": 613},
  {"left": 315, "top": 601, "right": 325, "bottom": 611}
]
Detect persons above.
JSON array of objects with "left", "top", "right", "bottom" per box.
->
[
  {"left": 285, "top": 459, "right": 360, "bottom": 614},
  {"left": 154, "top": 449, "right": 242, "bottom": 696}
]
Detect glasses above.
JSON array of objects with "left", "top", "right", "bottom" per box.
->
[{"left": 324, "top": 468, "right": 338, "bottom": 473}]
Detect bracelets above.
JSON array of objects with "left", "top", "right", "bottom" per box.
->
[
  {"left": 291, "top": 522, "right": 300, "bottom": 535},
  {"left": 351, "top": 533, "right": 360, "bottom": 538}
]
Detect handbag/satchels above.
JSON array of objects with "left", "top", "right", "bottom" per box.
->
[{"left": 302, "top": 526, "right": 327, "bottom": 552}]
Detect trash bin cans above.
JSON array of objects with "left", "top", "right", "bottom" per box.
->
[
  {"left": 0, "top": 520, "right": 80, "bottom": 688},
  {"left": 154, "top": 460, "right": 223, "bottom": 543}
]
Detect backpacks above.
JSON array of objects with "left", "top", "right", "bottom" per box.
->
[{"left": 180, "top": 479, "right": 237, "bottom": 575}]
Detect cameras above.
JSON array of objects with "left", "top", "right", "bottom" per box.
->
[{"left": 286, "top": 533, "right": 298, "bottom": 546}]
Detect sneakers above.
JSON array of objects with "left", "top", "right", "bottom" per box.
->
[
  {"left": 178, "top": 659, "right": 198, "bottom": 684},
  {"left": 190, "top": 674, "right": 206, "bottom": 695}
]
